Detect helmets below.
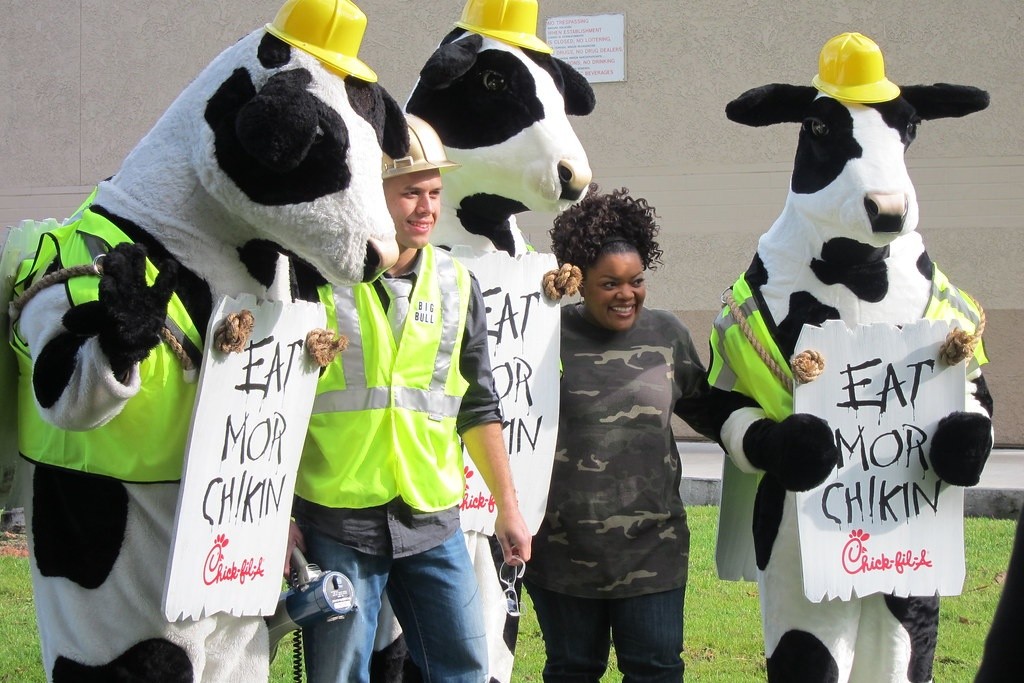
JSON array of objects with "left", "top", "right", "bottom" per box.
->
[
  {"left": 264, "top": 0, "right": 378, "bottom": 84},
  {"left": 454, "top": 0, "right": 553, "bottom": 54},
  {"left": 812, "top": 32, "right": 901, "bottom": 104},
  {"left": 380, "top": 112, "right": 462, "bottom": 179}
]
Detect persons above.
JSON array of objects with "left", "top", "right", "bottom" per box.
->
[
  {"left": 281, "top": 115, "right": 532, "bottom": 683},
  {"left": 522, "top": 194, "right": 707, "bottom": 683}
]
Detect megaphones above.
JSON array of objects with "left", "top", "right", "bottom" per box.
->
[{"left": 261, "top": 545, "right": 356, "bottom": 666}]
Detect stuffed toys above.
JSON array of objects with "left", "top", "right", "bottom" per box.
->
[
  {"left": 370, "top": 0, "right": 598, "bottom": 683},
  {"left": 706, "top": 30, "right": 995, "bottom": 683},
  {"left": 1, "top": 0, "right": 409, "bottom": 682}
]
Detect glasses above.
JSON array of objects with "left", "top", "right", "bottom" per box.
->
[{"left": 499, "top": 556, "right": 526, "bottom": 618}]
